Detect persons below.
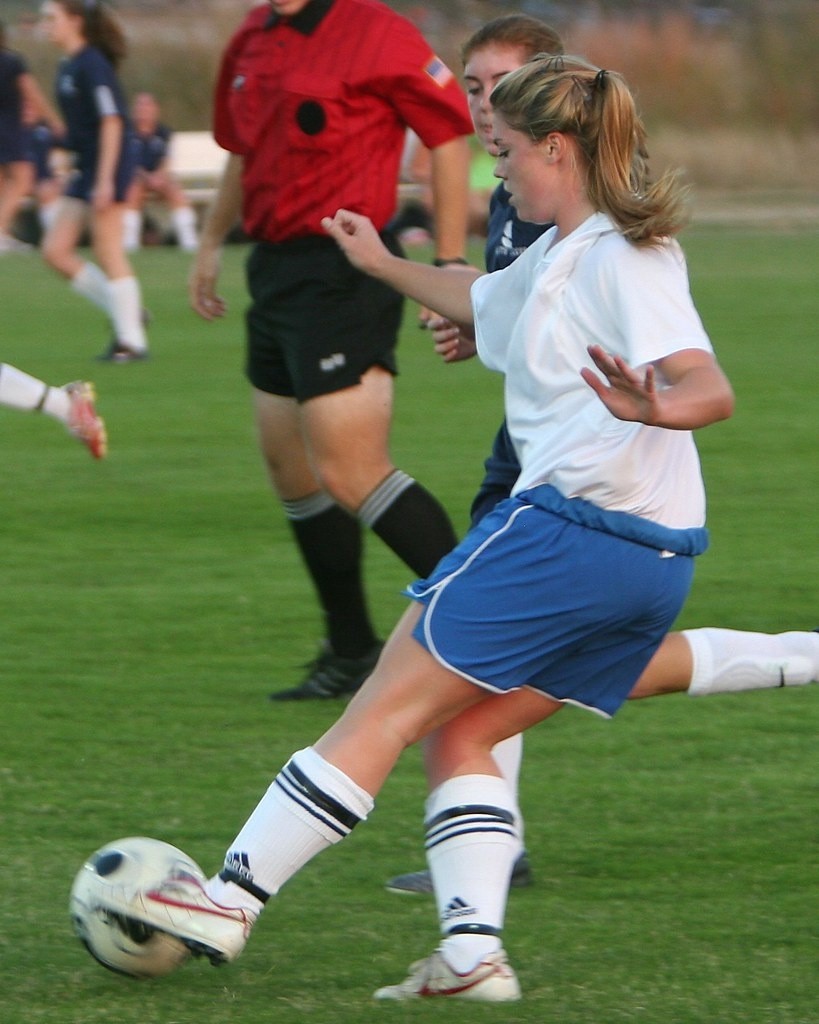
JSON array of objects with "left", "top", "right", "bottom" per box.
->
[
  {"left": 99, "top": 54, "right": 733, "bottom": 1001},
  {"left": 189, "top": 0, "right": 475, "bottom": 703},
  {"left": 123, "top": 92, "right": 198, "bottom": 248},
  {"left": 0, "top": 364, "right": 107, "bottom": 459},
  {"left": 382, "top": 16, "right": 819, "bottom": 893},
  {"left": 0, "top": 20, "right": 67, "bottom": 258},
  {"left": 41, "top": 0, "right": 152, "bottom": 364}
]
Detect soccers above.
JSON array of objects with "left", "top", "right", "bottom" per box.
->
[{"left": 68, "top": 836, "right": 209, "bottom": 981}]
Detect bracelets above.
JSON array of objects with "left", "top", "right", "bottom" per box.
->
[{"left": 434, "top": 258, "right": 468, "bottom": 267}]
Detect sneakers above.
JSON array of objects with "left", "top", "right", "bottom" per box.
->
[
  {"left": 61, "top": 380, "right": 107, "bottom": 458},
  {"left": 269, "top": 643, "right": 386, "bottom": 701},
  {"left": 383, "top": 848, "right": 535, "bottom": 898},
  {"left": 372, "top": 949, "right": 521, "bottom": 1002},
  {"left": 92, "top": 873, "right": 256, "bottom": 967}
]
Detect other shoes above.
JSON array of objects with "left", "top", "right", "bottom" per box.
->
[{"left": 106, "top": 311, "right": 149, "bottom": 365}]
